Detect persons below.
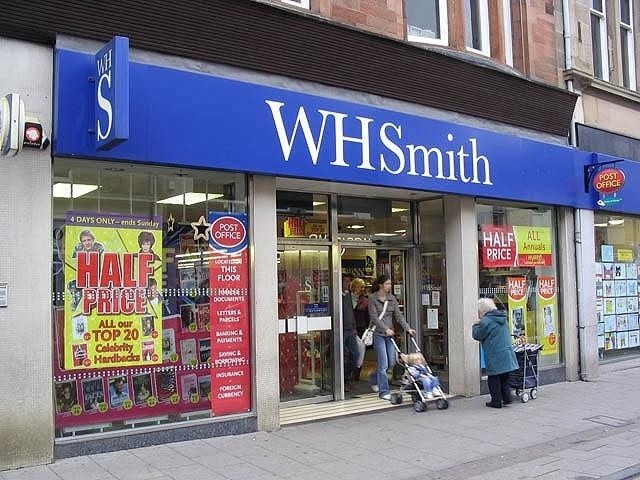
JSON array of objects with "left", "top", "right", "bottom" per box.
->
[
  {"left": 111, "top": 377, "right": 128, "bottom": 408},
  {"left": 72, "top": 229, "right": 105, "bottom": 254},
  {"left": 513, "top": 308, "right": 526, "bottom": 339},
  {"left": 309, "top": 271, "right": 370, "bottom": 393},
  {"left": 61, "top": 382, "right": 77, "bottom": 412},
  {"left": 471, "top": 297, "right": 520, "bottom": 409},
  {"left": 367, "top": 274, "right": 417, "bottom": 401},
  {"left": 407, "top": 352, "right": 442, "bottom": 399},
  {"left": 68, "top": 280, "right": 83, "bottom": 312},
  {"left": 544, "top": 306, "right": 553, "bottom": 336},
  {"left": 136, "top": 230, "right": 162, "bottom": 262},
  {"left": 146, "top": 278, "right": 161, "bottom": 307}
]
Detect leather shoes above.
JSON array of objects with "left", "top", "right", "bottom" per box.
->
[{"left": 486, "top": 400, "right": 502, "bottom": 408}]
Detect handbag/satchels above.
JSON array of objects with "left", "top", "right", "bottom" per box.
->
[{"left": 362, "top": 329, "right": 373, "bottom": 346}]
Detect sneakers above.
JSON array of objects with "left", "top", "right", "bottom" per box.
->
[
  {"left": 382, "top": 394, "right": 391, "bottom": 400},
  {"left": 371, "top": 385, "right": 379, "bottom": 393}
]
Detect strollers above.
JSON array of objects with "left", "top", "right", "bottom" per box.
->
[{"left": 385, "top": 330, "right": 449, "bottom": 415}]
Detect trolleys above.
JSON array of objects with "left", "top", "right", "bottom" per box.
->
[{"left": 502, "top": 330, "right": 544, "bottom": 403}]
